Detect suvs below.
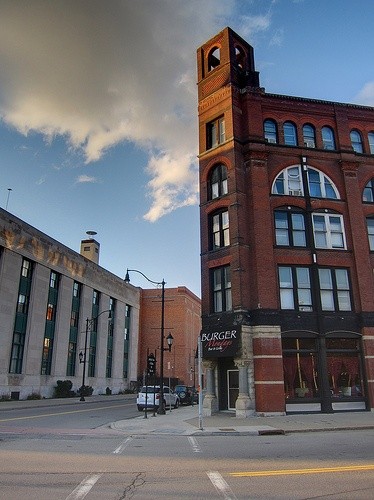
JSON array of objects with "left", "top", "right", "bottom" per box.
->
[
  {"left": 173, "top": 384, "right": 199, "bottom": 406},
  {"left": 136, "top": 385, "right": 180, "bottom": 412}
]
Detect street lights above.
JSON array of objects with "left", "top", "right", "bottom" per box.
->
[
  {"left": 122, "top": 267, "right": 174, "bottom": 415},
  {"left": 79, "top": 309, "right": 113, "bottom": 401}
]
